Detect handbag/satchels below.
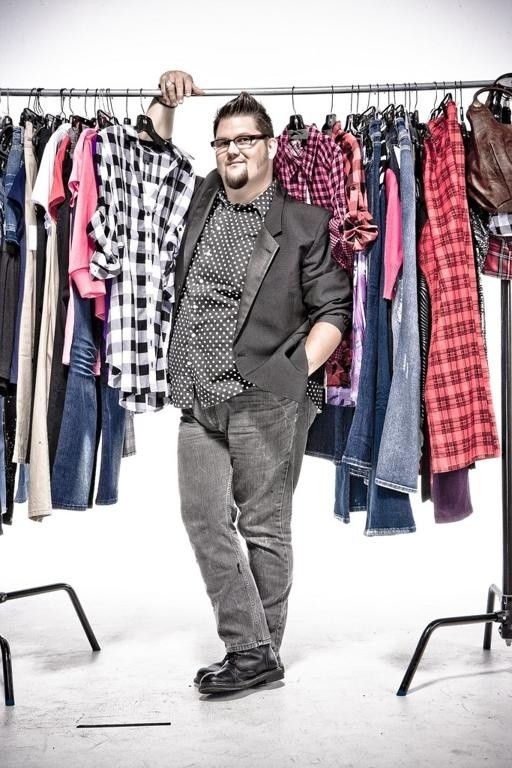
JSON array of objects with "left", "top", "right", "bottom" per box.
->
[{"left": 465, "top": 99, "right": 512, "bottom": 213}]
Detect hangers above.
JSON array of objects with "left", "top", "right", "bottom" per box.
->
[
  {"left": 0, "top": 85, "right": 169, "bottom": 152},
  {"left": 282, "top": 79, "right": 471, "bottom": 150}
]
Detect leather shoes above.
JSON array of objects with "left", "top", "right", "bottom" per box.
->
[{"left": 194, "top": 643, "right": 284, "bottom": 694}]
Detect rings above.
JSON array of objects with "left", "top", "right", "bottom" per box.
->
[{"left": 165, "top": 79, "right": 175, "bottom": 90}]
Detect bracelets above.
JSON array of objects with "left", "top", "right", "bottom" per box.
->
[{"left": 154, "top": 96, "right": 178, "bottom": 108}]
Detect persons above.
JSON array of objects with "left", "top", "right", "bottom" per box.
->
[{"left": 136, "top": 71, "right": 352, "bottom": 694}]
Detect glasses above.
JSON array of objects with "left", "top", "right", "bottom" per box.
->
[{"left": 210, "top": 134, "right": 270, "bottom": 149}]
